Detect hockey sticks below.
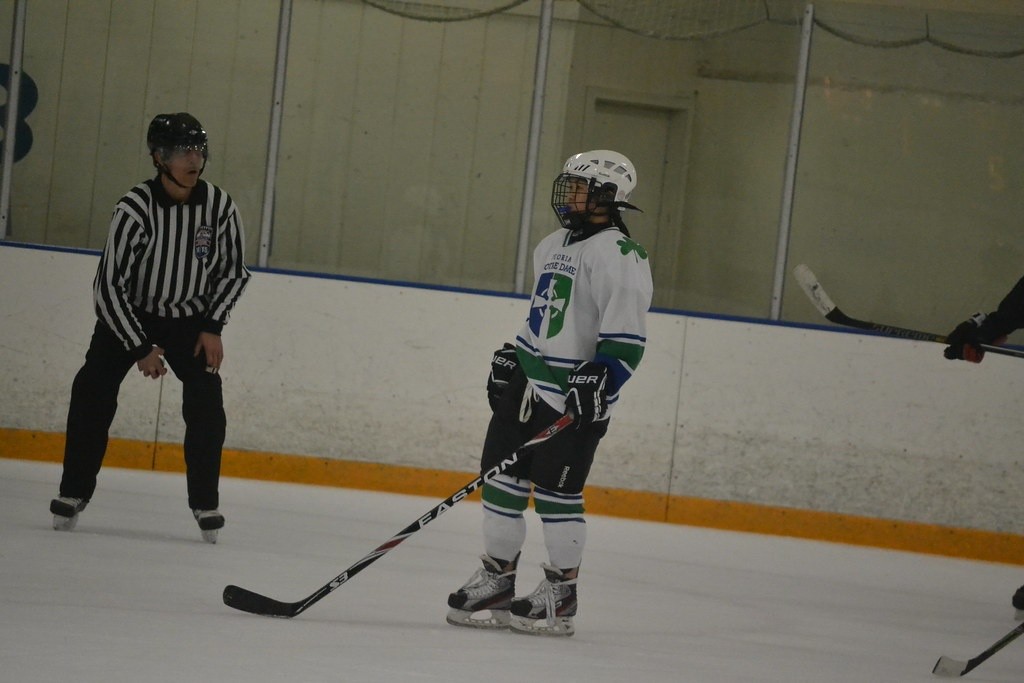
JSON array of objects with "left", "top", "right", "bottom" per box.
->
[
  {"left": 932, "top": 620, "right": 1024, "bottom": 677},
  {"left": 221, "top": 415, "right": 581, "bottom": 618},
  {"left": 795, "top": 261, "right": 1024, "bottom": 358}
]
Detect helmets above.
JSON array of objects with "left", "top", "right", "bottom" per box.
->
[
  {"left": 146, "top": 111, "right": 207, "bottom": 146},
  {"left": 551, "top": 149, "right": 638, "bottom": 229}
]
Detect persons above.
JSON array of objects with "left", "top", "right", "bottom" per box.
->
[
  {"left": 48, "top": 112, "right": 251, "bottom": 544},
  {"left": 446, "top": 148, "right": 653, "bottom": 636},
  {"left": 942, "top": 278, "right": 1024, "bottom": 620}
]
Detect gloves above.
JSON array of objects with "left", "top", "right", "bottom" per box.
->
[
  {"left": 562, "top": 359, "right": 614, "bottom": 430},
  {"left": 486, "top": 342, "right": 519, "bottom": 413},
  {"left": 944, "top": 308, "right": 1009, "bottom": 364}
]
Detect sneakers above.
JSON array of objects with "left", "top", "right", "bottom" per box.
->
[
  {"left": 445, "top": 550, "right": 520, "bottom": 628},
  {"left": 507, "top": 562, "right": 580, "bottom": 633},
  {"left": 190, "top": 508, "right": 225, "bottom": 544},
  {"left": 48, "top": 495, "right": 91, "bottom": 531}
]
